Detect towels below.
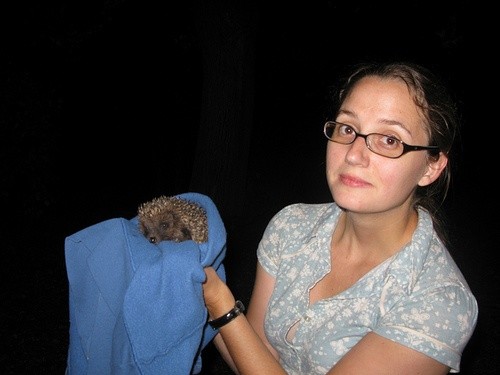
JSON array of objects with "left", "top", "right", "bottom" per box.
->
[{"left": 65, "top": 191, "right": 228, "bottom": 374}]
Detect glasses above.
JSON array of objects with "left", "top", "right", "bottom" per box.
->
[{"left": 324, "top": 120, "right": 440, "bottom": 158}]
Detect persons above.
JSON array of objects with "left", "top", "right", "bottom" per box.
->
[{"left": 201, "top": 54, "right": 481, "bottom": 375}]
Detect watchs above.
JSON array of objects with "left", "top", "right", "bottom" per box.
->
[{"left": 208, "top": 300, "right": 245, "bottom": 330}]
